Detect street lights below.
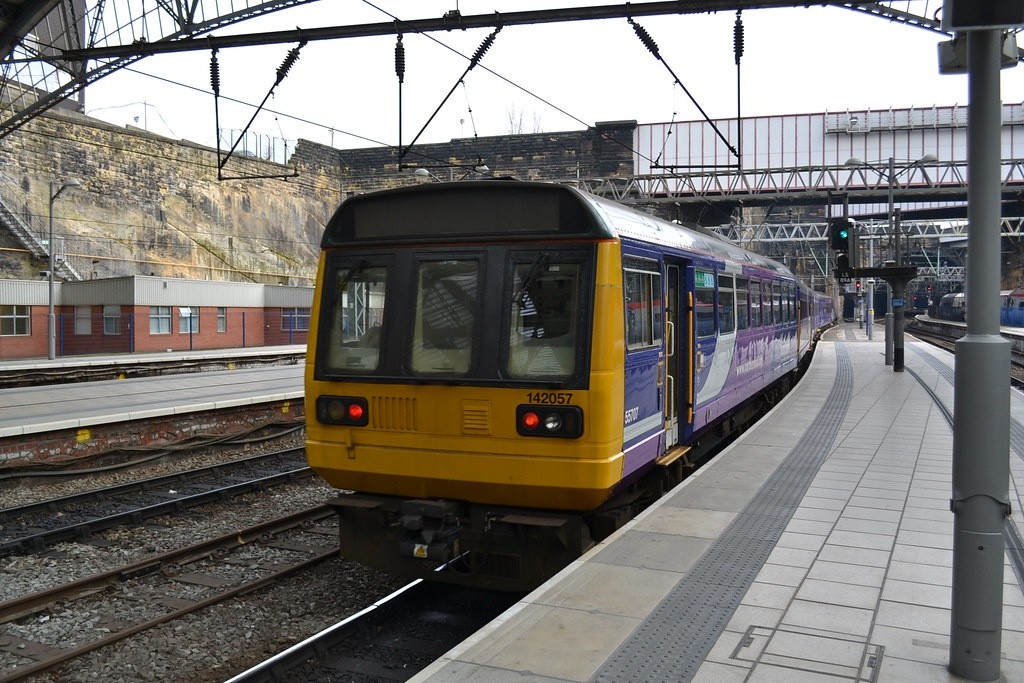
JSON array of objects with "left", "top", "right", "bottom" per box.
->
[
  {"left": 48, "top": 179, "right": 81, "bottom": 361},
  {"left": 846, "top": 154, "right": 940, "bottom": 366}
]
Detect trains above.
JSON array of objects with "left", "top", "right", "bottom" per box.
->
[
  {"left": 939, "top": 286, "right": 1024, "bottom": 323},
  {"left": 302, "top": 177, "right": 849, "bottom": 592}
]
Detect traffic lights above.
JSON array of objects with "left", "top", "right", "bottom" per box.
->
[
  {"left": 856, "top": 280, "right": 861, "bottom": 289},
  {"left": 838, "top": 254, "right": 851, "bottom": 283},
  {"left": 831, "top": 219, "right": 850, "bottom": 250}
]
